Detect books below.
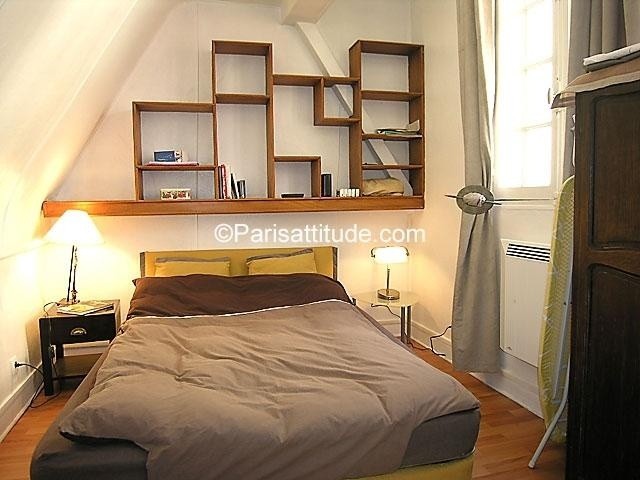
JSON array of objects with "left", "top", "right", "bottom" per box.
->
[
  {"left": 56, "top": 299, "right": 113, "bottom": 316},
  {"left": 218, "top": 164, "right": 241, "bottom": 199}
]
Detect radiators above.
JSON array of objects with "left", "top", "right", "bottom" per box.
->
[{"left": 500, "top": 236, "right": 553, "bottom": 367}]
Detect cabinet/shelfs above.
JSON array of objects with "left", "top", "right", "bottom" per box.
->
[
  {"left": 562, "top": 49, "right": 639, "bottom": 480},
  {"left": 130, "top": 37, "right": 426, "bottom": 218}
]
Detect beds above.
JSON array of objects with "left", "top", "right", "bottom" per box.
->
[{"left": 28, "top": 244, "right": 484, "bottom": 479}]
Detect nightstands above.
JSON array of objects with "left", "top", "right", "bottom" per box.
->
[{"left": 39, "top": 297, "right": 122, "bottom": 396}]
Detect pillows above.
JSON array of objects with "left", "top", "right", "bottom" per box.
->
[
  {"left": 154, "top": 256, "right": 231, "bottom": 279},
  {"left": 245, "top": 247, "right": 318, "bottom": 277}
]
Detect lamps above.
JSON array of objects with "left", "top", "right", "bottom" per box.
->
[
  {"left": 44, "top": 210, "right": 105, "bottom": 308},
  {"left": 369, "top": 244, "right": 410, "bottom": 301}
]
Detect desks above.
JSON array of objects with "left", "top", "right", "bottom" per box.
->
[
  {"left": 528, "top": 174, "right": 575, "bottom": 469},
  {"left": 352, "top": 290, "right": 420, "bottom": 344}
]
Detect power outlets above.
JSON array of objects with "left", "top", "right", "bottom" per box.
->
[
  {"left": 9, "top": 356, "right": 20, "bottom": 375},
  {"left": 447, "top": 322, "right": 452, "bottom": 332}
]
